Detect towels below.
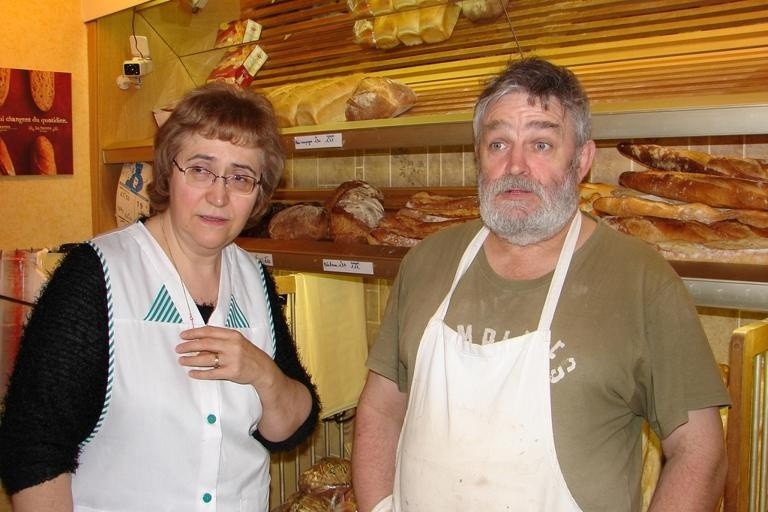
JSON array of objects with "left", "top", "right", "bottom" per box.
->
[{"left": 285, "top": 271, "right": 371, "bottom": 420}]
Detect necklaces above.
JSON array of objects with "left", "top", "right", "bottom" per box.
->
[{"left": 158, "top": 214, "right": 197, "bottom": 330}]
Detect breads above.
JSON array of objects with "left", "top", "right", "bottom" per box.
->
[
  {"left": 28, "top": 135, "right": 57, "bottom": 175},
  {"left": 264, "top": 73, "right": 418, "bottom": 130},
  {"left": 283, "top": 456, "right": 358, "bottom": 512},
  {"left": 0, "top": 138, "right": 16, "bottom": 176},
  {"left": 578, "top": 143, "right": 768, "bottom": 265},
  {"left": 28, "top": 70, "right": 55, "bottom": 112},
  {"left": 0, "top": 68, "right": 11, "bottom": 106},
  {"left": 348, "top": 1, "right": 508, "bottom": 50},
  {"left": 268, "top": 181, "right": 481, "bottom": 248}
]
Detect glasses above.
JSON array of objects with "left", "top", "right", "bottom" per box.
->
[{"left": 168, "top": 157, "right": 262, "bottom": 194}]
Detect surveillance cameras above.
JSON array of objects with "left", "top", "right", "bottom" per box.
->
[{"left": 122, "top": 36, "right": 152, "bottom": 78}]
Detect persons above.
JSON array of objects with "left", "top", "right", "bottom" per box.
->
[
  {"left": 349, "top": 57, "right": 735, "bottom": 511},
  {"left": 0, "top": 76, "right": 323, "bottom": 512}
]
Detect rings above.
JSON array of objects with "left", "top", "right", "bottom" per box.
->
[{"left": 213, "top": 352, "right": 219, "bottom": 368}]
